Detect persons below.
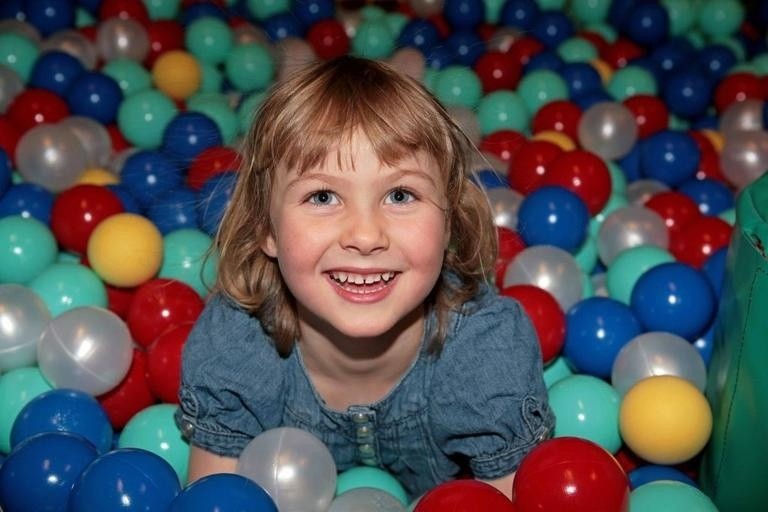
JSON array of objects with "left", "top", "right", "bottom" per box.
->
[{"left": 174, "top": 54, "right": 558, "bottom": 503}]
